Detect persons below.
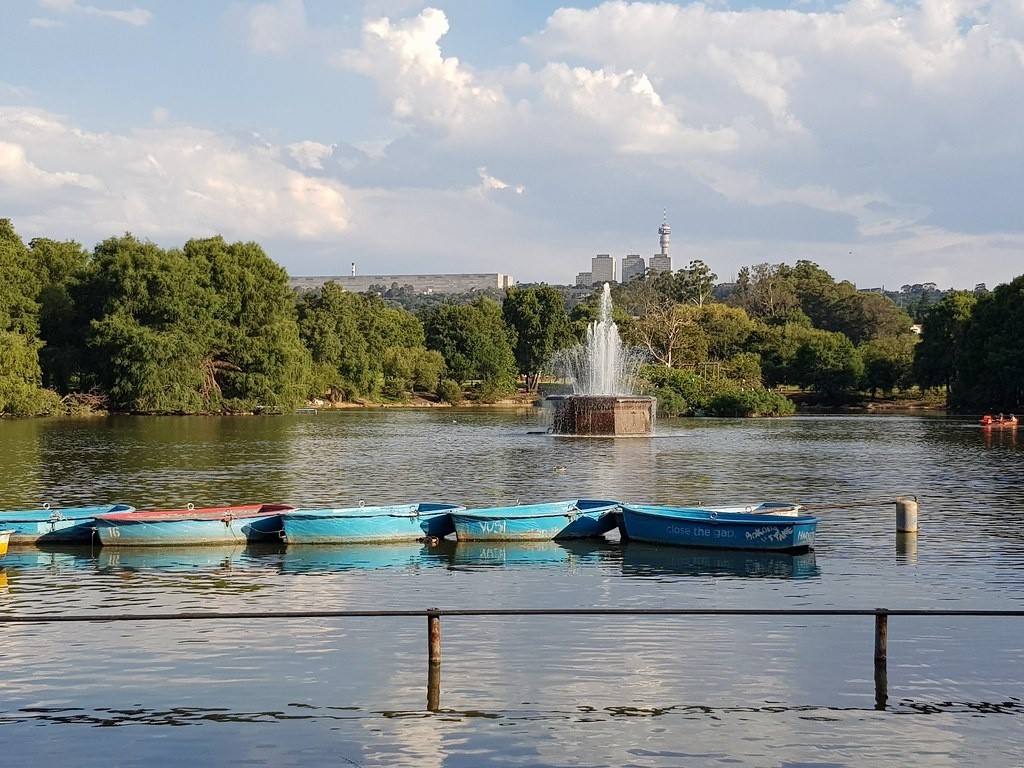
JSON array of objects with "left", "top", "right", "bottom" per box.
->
[
  {"left": 1010, "top": 414, "right": 1015, "bottom": 421},
  {"left": 996, "top": 413, "right": 1003, "bottom": 421},
  {"left": 983, "top": 413, "right": 992, "bottom": 420}
]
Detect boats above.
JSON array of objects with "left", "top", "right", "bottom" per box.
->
[
  {"left": 618, "top": 502, "right": 821, "bottom": 547},
  {"left": 978, "top": 419, "right": 1017, "bottom": 426},
  {"left": 91, "top": 503, "right": 297, "bottom": 546},
  {"left": 448, "top": 500, "right": 621, "bottom": 542},
  {"left": 0, "top": 505, "right": 136, "bottom": 541},
  {"left": 280, "top": 503, "right": 466, "bottom": 544}
]
